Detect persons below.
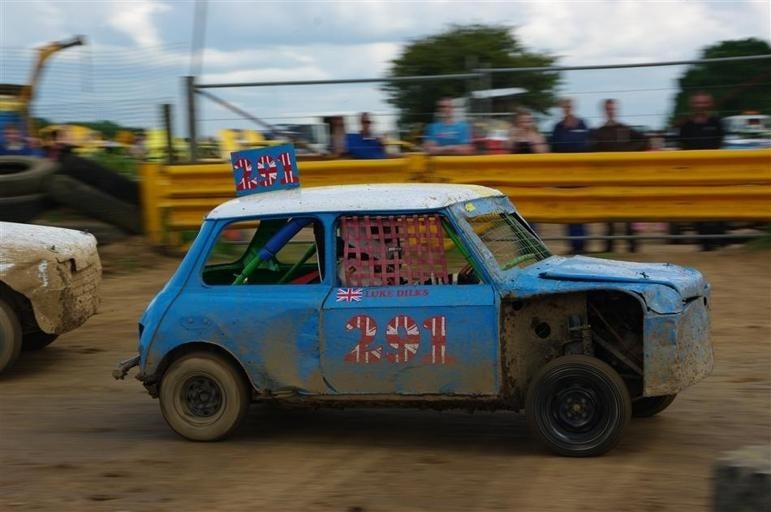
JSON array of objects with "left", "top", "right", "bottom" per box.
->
[
  {"left": 424, "top": 97, "right": 477, "bottom": 156},
  {"left": 508, "top": 108, "right": 551, "bottom": 153},
  {"left": 676, "top": 93, "right": 726, "bottom": 251},
  {"left": 552, "top": 99, "right": 648, "bottom": 254},
  {"left": 334, "top": 220, "right": 481, "bottom": 286}
]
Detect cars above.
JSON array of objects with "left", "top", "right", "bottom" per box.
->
[
  {"left": 0, "top": 221, "right": 103, "bottom": 374},
  {"left": 111, "top": 143, "right": 715, "bottom": 455},
  {"left": 721, "top": 110, "right": 770, "bottom": 140}
]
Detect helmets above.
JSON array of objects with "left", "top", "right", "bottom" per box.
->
[{"left": 339, "top": 217, "right": 401, "bottom": 265}]
